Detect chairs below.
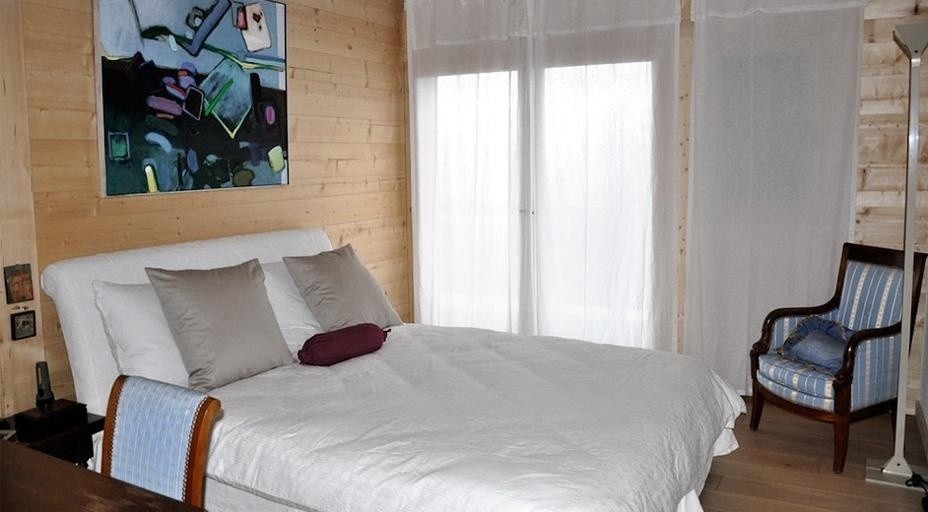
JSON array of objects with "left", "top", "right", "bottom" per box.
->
[
  {"left": 748, "top": 243, "right": 928, "bottom": 474},
  {"left": 100, "top": 375, "right": 221, "bottom": 508}
]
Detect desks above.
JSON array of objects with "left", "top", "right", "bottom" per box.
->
[{"left": 0, "top": 438, "right": 210, "bottom": 512}]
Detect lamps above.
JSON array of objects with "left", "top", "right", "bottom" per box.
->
[{"left": 865, "top": 23, "right": 928, "bottom": 492}]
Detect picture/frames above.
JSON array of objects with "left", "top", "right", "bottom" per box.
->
[{"left": 10, "top": 310, "right": 36, "bottom": 340}]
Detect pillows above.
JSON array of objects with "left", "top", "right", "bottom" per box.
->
[
  {"left": 92, "top": 280, "right": 189, "bottom": 386},
  {"left": 779, "top": 315, "right": 857, "bottom": 375},
  {"left": 282, "top": 243, "right": 404, "bottom": 333},
  {"left": 261, "top": 261, "right": 322, "bottom": 361},
  {"left": 298, "top": 324, "right": 391, "bottom": 366},
  {"left": 144, "top": 258, "right": 297, "bottom": 393}
]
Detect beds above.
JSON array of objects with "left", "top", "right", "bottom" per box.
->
[{"left": 39, "top": 227, "right": 747, "bottom": 512}]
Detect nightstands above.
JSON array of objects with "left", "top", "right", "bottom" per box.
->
[{"left": 0, "top": 412, "right": 105, "bottom": 469}]
